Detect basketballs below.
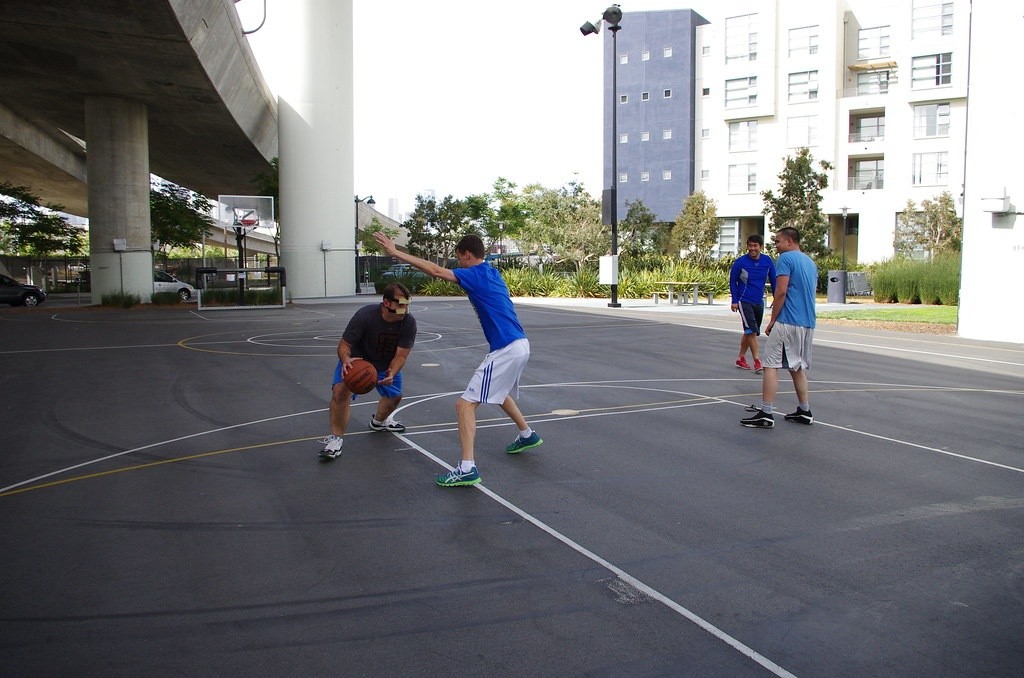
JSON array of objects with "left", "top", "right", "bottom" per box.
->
[{"left": 343, "top": 360, "right": 378, "bottom": 394}]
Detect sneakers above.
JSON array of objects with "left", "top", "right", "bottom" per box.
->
[
  {"left": 505, "top": 430, "right": 544, "bottom": 454},
  {"left": 754, "top": 359, "right": 763, "bottom": 372},
  {"left": 368, "top": 415, "right": 405, "bottom": 431},
  {"left": 740, "top": 405, "right": 775, "bottom": 428},
  {"left": 736, "top": 356, "right": 751, "bottom": 370},
  {"left": 435, "top": 461, "right": 481, "bottom": 486},
  {"left": 317, "top": 435, "right": 344, "bottom": 458},
  {"left": 785, "top": 407, "right": 814, "bottom": 425}
]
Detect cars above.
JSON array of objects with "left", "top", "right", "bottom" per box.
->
[
  {"left": 0, "top": 273, "right": 48, "bottom": 308},
  {"left": 153, "top": 270, "right": 195, "bottom": 302},
  {"left": 516, "top": 242, "right": 562, "bottom": 265}
]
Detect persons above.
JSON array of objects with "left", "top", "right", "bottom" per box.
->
[
  {"left": 730, "top": 234, "right": 776, "bottom": 371},
  {"left": 739, "top": 227, "right": 818, "bottom": 430},
  {"left": 318, "top": 282, "right": 417, "bottom": 459},
  {"left": 374, "top": 231, "right": 543, "bottom": 486}
]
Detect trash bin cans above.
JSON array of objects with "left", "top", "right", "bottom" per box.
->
[{"left": 827, "top": 270, "right": 846, "bottom": 303}]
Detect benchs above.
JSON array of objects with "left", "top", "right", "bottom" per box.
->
[{"left": 650, "top": 289, "right": 768, "bottom": 307}]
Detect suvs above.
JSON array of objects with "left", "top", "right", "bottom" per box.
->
[{"left": 67, "top": 263, "right": 90, "bottom": 271}]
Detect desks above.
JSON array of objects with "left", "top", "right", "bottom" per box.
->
[{"left": 655, "top": 282, "right": 700, "bottom": 306}]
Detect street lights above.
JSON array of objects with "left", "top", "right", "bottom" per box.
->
[
  {"left": 579, "top": 3, "right": 623, "bottom": 308},
  {"left": 497, "top": 220, "right": 505, "bottom": 274},
  {"left": 839, "top": 205, "right": 851, "bottom": 270},
  {"left": 354, "top": 194, "right": 376, "bottom": 293}
]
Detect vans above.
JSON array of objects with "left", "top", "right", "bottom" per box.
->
[{"left": 382, "top": 263, "right": 429, "bottom": 281}]
